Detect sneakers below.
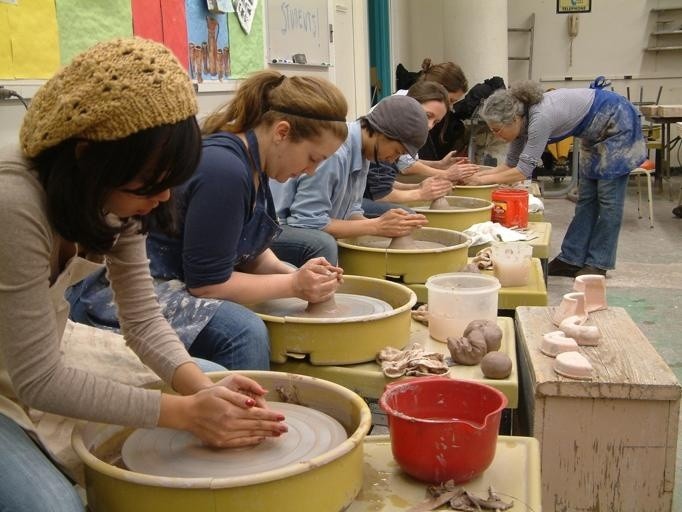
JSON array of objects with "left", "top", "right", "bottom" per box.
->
[
  {"left": 547, "top": 256, "right": 580, "bottom": 278},
  {"left": 572, "top": 263, "right": 608, "bottom": 282}
]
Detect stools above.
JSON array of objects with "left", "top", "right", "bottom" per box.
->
[
  {"left": 533, "top": 84, "right": 663, "bottom": 228},
  {"left": 514, "top": 304, "right": 682, "bottom": 512}
]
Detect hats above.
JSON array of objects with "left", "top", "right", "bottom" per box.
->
[
  {"left": 364, "top": 92, "right": 430, "bottom": 163},
  {"left": 18, "top": 34, "right": 200, "bottom": 161}
]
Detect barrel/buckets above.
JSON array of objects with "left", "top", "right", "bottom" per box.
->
[
  {"left": 426, "top": 272, "right": 502, "bottom": 343},
  {"left": 492, "top": 189, "right": 529, "bottom": 227},
  {"left": 493, "top": 242, "right": 534, "bottom": 287}
]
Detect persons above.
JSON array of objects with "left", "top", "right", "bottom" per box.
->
[
  {"left": 0, "top": 36, "right": 291, "bottom": 511},
  {"left": 455, "top": 78, "right": 647, "bottom": 277},
  {"left": 266, "top": 94, "right": 429, "bottom": 268},
  {"left": 368, "top": 58, "right": 482, "bottom": 184},
  {"left": 364, "top": 81, "right": 454, "bottom": 206},
  {"left": 63, "top": 66, "right": 347, "bottom": 370}
]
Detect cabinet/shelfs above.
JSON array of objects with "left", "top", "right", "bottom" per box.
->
[{"left": 644, "top": 8, "right": 682, "bottom": 51}]
